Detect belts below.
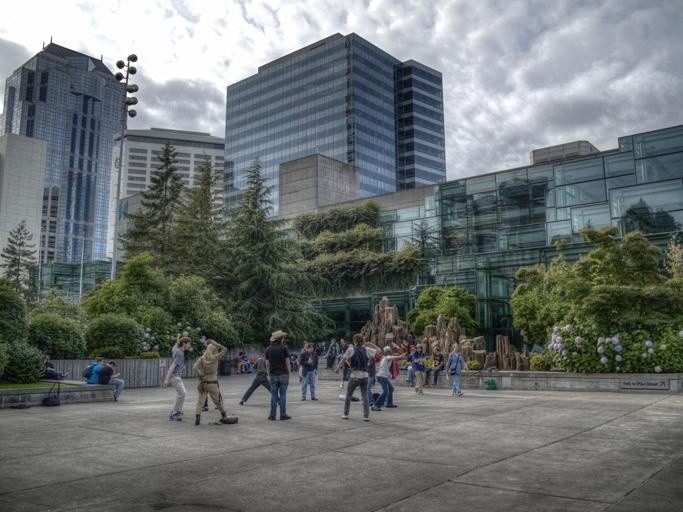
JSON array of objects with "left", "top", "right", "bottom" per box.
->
[{"left": 202, "top": 381, "right": 217, "bottom": 384}]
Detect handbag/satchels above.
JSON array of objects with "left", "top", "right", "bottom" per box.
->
[
  {"left": 43, "top": 382, "right": 60, "bottom": 407},
  {"left": 448, "top": 368, "right": 456, "bottom": 375}
]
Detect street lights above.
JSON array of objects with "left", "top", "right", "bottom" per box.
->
[{"left": 104, "top": 52, "right": 141, "bottom": 281}]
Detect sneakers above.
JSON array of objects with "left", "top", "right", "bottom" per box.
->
[
  {"left": 415, "top": 388, "right": 423, "bottom": 396},
  {"left": 268, "top": 416, "right": 292, "bottom": 420},
  {"left": 370, "top": 404, "right": 398, "bottom": 411},
  {"left": 341, "top": 415, "right": 369, "bottom": 421},
  {"left": 169, "top": 407, "right": 234, "bottom": 425},
  {"left": 240, "top": 401, "right": 244, "bottom": 404}
]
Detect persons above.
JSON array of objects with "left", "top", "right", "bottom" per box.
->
[
  {"left": 82, "top": 356, "right": 125, "bottom": 402},
  {"left": 161, "top": 327, "right": 468, "bottom": 428}
]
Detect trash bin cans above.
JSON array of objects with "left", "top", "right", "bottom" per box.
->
[{"left": 219, "top": 359, "right": 231, "bottom": 376}]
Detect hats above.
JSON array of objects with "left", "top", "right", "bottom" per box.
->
[{"left": 270, "top": 330, "right": 287, "bottom": 342}]
